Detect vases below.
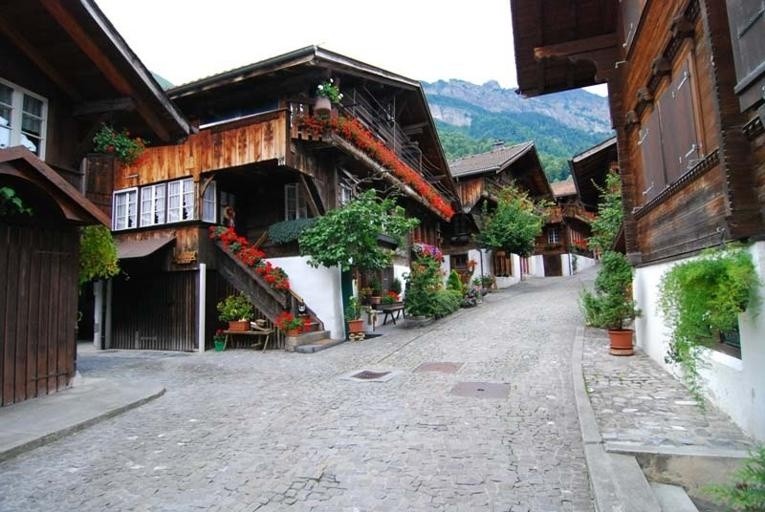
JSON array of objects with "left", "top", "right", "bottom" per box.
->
[
  {"left": 313, "top": 96, "right": 332, "bottom": 114},
  {"left": 288, "top": 329, "right": 299, "bottom": 336}
]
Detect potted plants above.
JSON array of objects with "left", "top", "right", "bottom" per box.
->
[
  {"left": 216, "top": 294, "right": 255, "bottom": 331},
  {"left": 605, "top": 274, "right": 642, "bottom": 351},
  {"left": 345, "top": 296, "right": 365, "bottom": 332},
  {"left": 369, "top": 276, "right": 400, "bottom": 304}
]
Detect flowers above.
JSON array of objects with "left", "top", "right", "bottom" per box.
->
[
  {"left": 208, "top": 223, "right": 289, "bottom": 292},
  {"left": 316, "top": 78, "right": 344, "bottom": 104},
  {"left": 95, "top": 125, "right": 151, "bottom": 166},
  {"left": 275, "top": 311, "right": 302, "bottom": 331}
]
